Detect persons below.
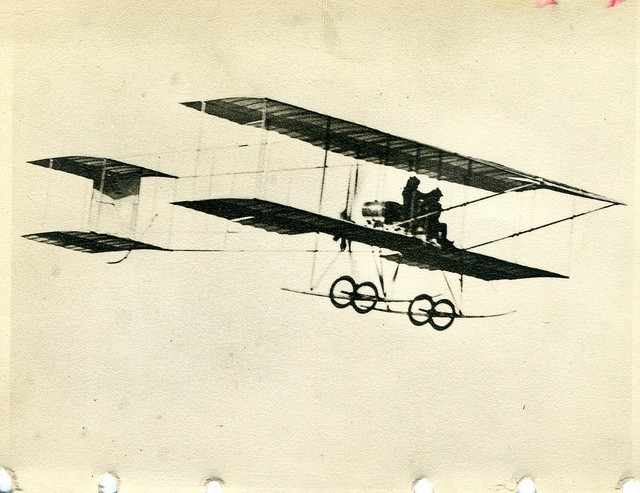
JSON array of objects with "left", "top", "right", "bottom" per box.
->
[{"left": 402, "top": 176, "right": 453, "bottom": 248}]
